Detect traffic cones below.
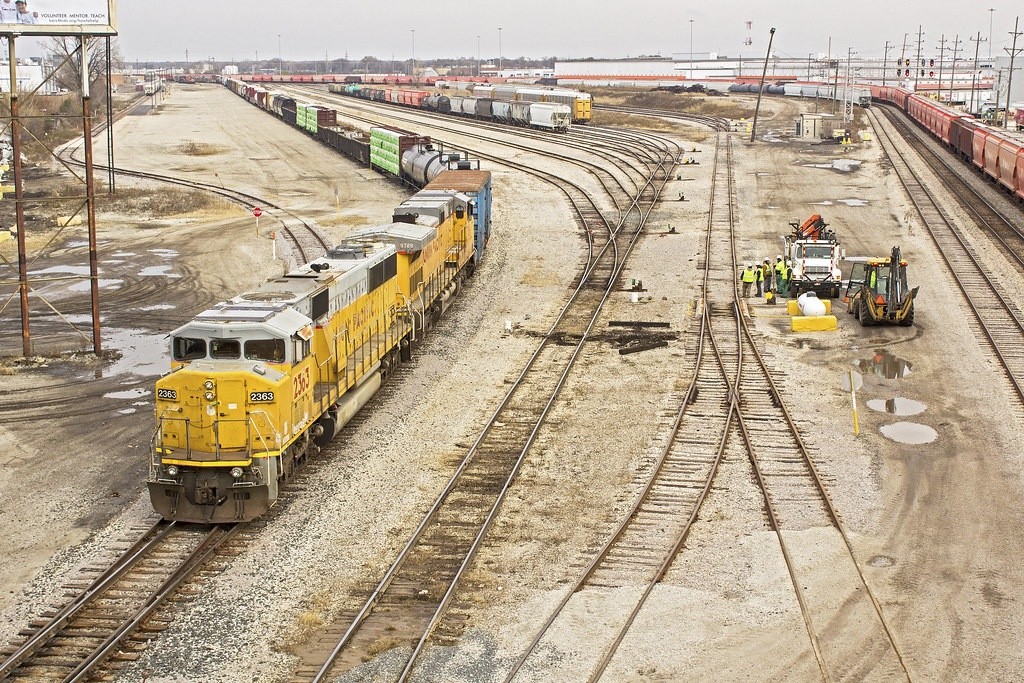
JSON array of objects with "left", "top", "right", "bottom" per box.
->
[
  {"left": 842, "top": 138, "right": 847, "bottom": 144},
  {"left": 863, "top": 129, "right": 872, "bottom": 141},
  {"left": 744, "top": 121, "right": 753, "bottom": 132},
  {"left": 846, "top": 137, "right": 852, "bottom": 144}
]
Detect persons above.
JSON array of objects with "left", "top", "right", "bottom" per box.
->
[
  {"left": 0, "top": 0, "right": 38, "bottom": 26},
  {"left": 739, "top": 255, "right": 792, "bottom": 297},
  {"left": 15, "top": 0, "right": 39, "bottom": 24}
]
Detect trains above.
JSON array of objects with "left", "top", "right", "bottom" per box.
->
[
  {"left": 793, "top": 76, "right": 1024, "bottom": 206},
  {"left": 785, "top": 82, "right": 872, "bottom": 109},
  {"left": 145, "top": 75, "right": 594, "bottom": 528}
]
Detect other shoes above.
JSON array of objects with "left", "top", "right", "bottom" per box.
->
[
  {"left": 781, "top": 295, "right": 787, "bottom": 298},
  {"left": 743, "top": 295, "right": 746, "bottom": 297},
  {"left": 755, "top": 294, "right": 761, "bottom": 297},
  {"left": 764, "top": 290, "right": 771, "bottom": 292},
  {"left": 747, "top": 295, "right": 750, "bottom": 298},
  {"left": 776, "top": 290, "right": 783, "bottom": 293}
]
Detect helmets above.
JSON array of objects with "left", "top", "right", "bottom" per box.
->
[
  {"left": 755, "top": 261, "right": 760, "bottom": 266},
  {"left": 787, "top": 260, "right": 792, "bottom": 266},
  {"left": 748, "top": 263, "right": 752, "bottom": 267},
  {"left": 777, "top": 254, "right": 782, "bottom": 260},
  {"left": 764, "top": 257, "right": 770, "bottom": 261}
]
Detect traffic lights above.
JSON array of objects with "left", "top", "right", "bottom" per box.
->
[{"left": 896, "top": 56, "right": 935, "bottom": 78}]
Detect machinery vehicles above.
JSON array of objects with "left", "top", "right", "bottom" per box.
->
[
  {"left": 784, "top": 214, "right": 849, "bottom": 297},
  {"left": 846, "top": 247, "right": 922, "bottom": 327}
]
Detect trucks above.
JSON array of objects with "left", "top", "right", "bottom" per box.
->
[{"left": 949, "top": 99, "right": 1024, "bottom": 134}]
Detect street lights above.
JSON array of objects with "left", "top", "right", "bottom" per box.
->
[
  {"left": 498, "top": 27, "right": 502, "bottom": 76},
  {"left": 477, "top": 35, "right": 481, "bottom": 76},
  {"left": 411, "top": 29, "right": 416, "bottom": 75},
  {"left": 689, "top": 19, "right": 696, "bottom": 78},
  {"left": 278, "top": 34, "right": 282, "bottom": 75},
  {"left": 987, "top": 7, "right": 996, "bottom": 82}
]
